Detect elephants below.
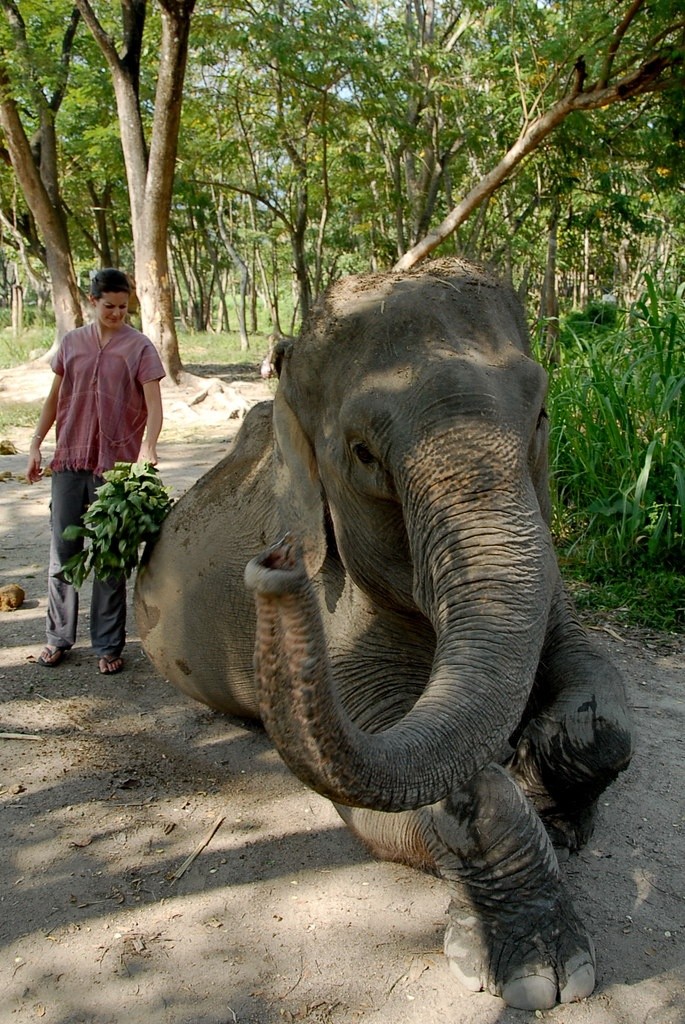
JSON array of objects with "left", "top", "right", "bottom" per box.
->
[{"left": 131, "top": 256, "right": 635, "bottom": 1011}]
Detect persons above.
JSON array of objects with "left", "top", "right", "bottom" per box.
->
[{"left": 27, "top": 268, "right": 166, "bottom": 676}]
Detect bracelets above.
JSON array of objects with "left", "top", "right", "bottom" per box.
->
[{"left": 31, "top": 434, "right": 43, "bottom": 442}]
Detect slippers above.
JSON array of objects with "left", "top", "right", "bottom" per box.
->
[
  {"left": 38, "top": 643, "right": 65, "bottom": 666},
  {"left": 98, "top": 654, "right": 125, "bottom": 675}
]
